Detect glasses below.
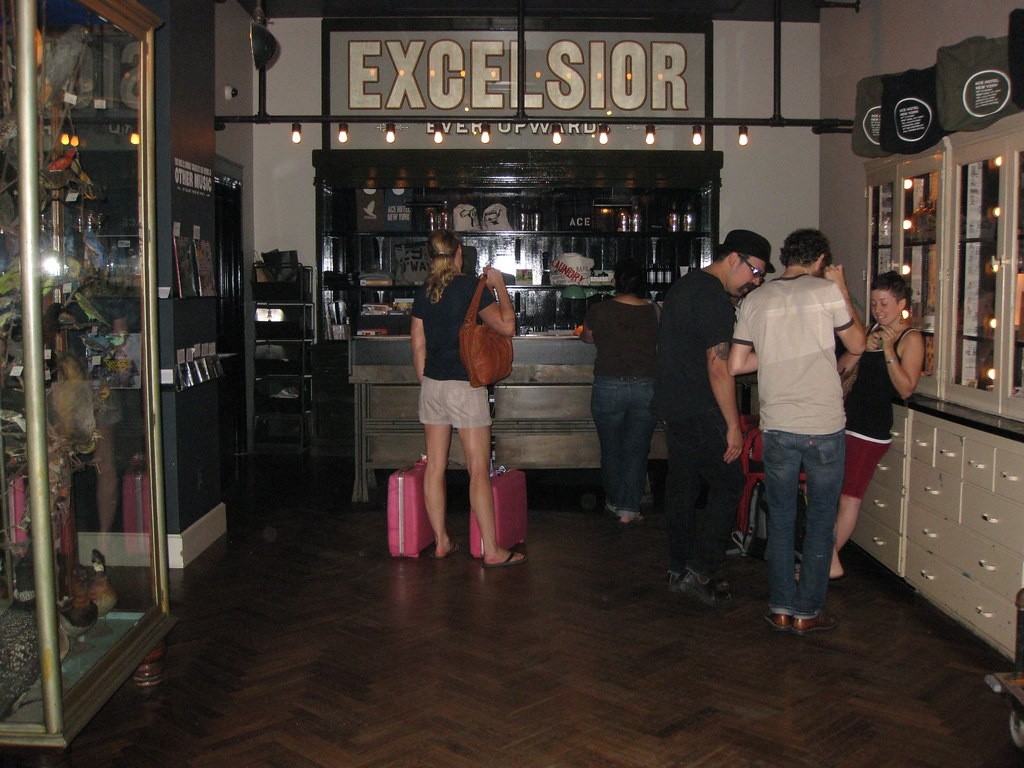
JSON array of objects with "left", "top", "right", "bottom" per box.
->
[{"left": 741, "top": 255, "right": 765, "bottom": 281}]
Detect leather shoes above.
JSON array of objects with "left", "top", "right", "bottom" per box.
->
[
  {"left": 791, "top": 612, "right": 839, "bottom": 632},
  {"left": 768, "top": 613, "right": 790, "bottom": 628}
]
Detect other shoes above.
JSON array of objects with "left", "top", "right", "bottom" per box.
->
[{"left": 676, "top": 570, "right": 739, "bottom": 610}]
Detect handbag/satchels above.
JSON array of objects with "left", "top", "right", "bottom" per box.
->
[
  {"left": 734, "top": 425, "right": 809, "bottom": 565},
  {"left": 458, "top": 273, "right": 513, "bottom": 388}
]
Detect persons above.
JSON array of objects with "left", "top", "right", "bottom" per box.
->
[
  {"left": 650, "top": 227, "right": 926, "bottom": 634},
  {"left": 40, "top": 245, "right": 130, "bottom": 533},
  {"left": 579, "top": 256, "right": 665, "bottom": 528},
  {"left": 410, "top": 228, "right": 528, "bottom": 568}
]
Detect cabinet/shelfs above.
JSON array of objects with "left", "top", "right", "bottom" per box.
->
[
  {"left": 846, "top": 110, "right": 1022, "bottom": 661},
  {"left": 352, "top": 226, "right": 708, "bottom": 290},
  {"left": 254, "top": 263, "right": 311, "bottom": 469}
]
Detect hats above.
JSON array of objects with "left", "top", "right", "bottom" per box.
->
[{"left": 717, "top": 229, "right": 775, "bottom": 274}]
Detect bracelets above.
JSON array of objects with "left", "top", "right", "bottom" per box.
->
[{"left": 886, "top": 357, "right": 897, "bottom": 364}]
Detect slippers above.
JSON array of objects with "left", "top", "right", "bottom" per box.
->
[
  {"left": 429, "top": 542, "right": 458, "bottom": 561},
  {"left": 605, "top": 504, "right": 645, "bottom": 529},
  {"left": 481, "top": 550, "right": 527, "bottom": 567}
]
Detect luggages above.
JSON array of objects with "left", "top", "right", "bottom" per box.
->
[
  {"left": 387, "top": 460, "right": 435, "bottom": 558},
  {"left": 468, "top": 450, "right": 526, "bottom": 558},
  {"left": 123, "top": 457, "right": 152, "bottom": 559},
  {"left": 6, "top": 458, "right": 76, "bottom": 564}
]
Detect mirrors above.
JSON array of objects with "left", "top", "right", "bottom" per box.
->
[
  {"left": 1011, "top": 152, "right": 1024, "bottom": 399},
  {"left": 902, "top": 169, "right": 939, "bottom": 380},
  {"left": 953, "top": 156, "right": 999, "bottom": 393}
]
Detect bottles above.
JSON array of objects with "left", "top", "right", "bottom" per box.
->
[
  {"left": 631, "top": 206, "right": 642, "bottom": 232},
  {"left": 617, "top": 207, "right": 631, "bottom": 232},
  {"left": 682, "top": 205, "right": 696, "bottom": 232},
  {"left": 425, "top": 212, "right": 438, "bottom": 231},
  {"left": 646, "top": 264, "right": 672, "bottom": 286},
  {"left": 519, "top": 203, "right": 543, "bottom": 231},
  {"left": 440, "top": 200, "right": 451, "bottom": 230},
  {"left": 667, "top": 205, "right": 680, "bottom": 232}
]
